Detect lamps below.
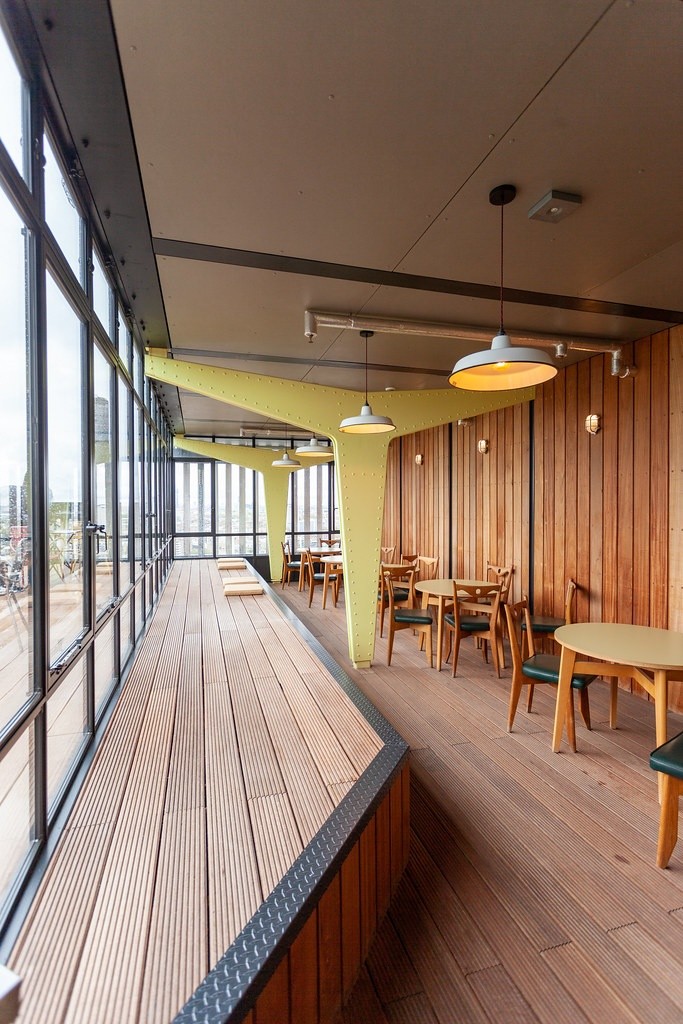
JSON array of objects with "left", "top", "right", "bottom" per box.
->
[
  {"left": 272, "top": 422, "right": 301, "bottom": 467},
  {"left": 585, "top": 414, "right": 601, "bottom": 435},
  {"left": 415, "top": 454, "right": 423, "bottom": 465},
  {"left": 477, "top": 440, "right": 488, "bottom": 455},
  {"left": 294, "top": 431, "right": 334, "bottom": 457},
  {"left": 337, "top": 329, "right": 396, "bottom": 435},
  {"left": 447, "top": 183, "right": 560, "bottom": 392}
]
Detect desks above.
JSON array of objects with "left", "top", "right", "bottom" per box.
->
[
  {"left": 551, "top": 623, "right": 683, "bottom": 805},
  {"left": 414, "top": 579, "right": 500, "bottom": 673},
  {"left": 319, "top": 555, "right": 343, "bottom": 610},
  {"left": 296, "top": 547, "right": 340, "bottom": 592}
]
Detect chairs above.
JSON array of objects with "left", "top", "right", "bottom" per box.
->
[
  {"left": 649, "top": 731, "right": 683, "bottom": 870},
  {"left": 0, "top": 536, "right": 31, "bottom": 654},
  {"left": 281, "top": 538, "right": 599, "bottom": 754}
]
